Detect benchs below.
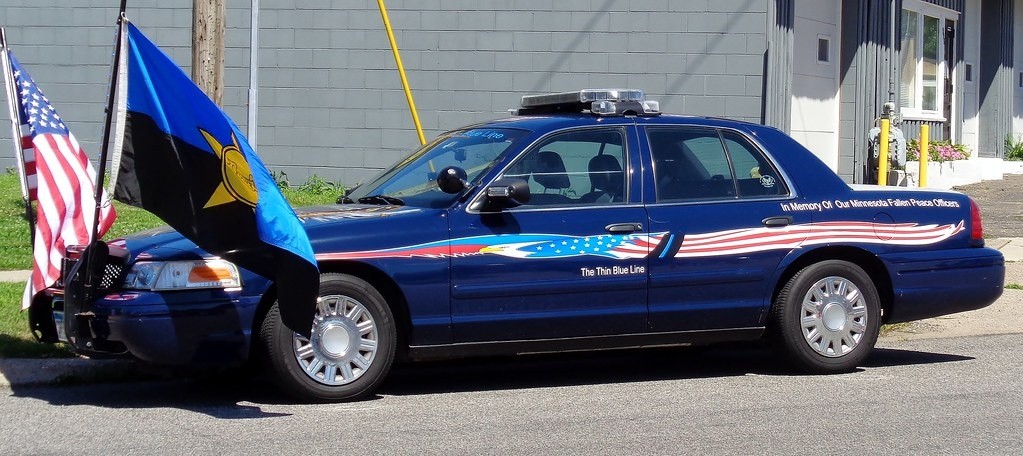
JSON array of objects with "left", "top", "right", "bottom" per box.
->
[{"left": 681, "top": 175, "right": 775, "bottom": 196}]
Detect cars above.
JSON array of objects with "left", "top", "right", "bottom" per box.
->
[{"left": 28, "top": 90, "right": 1005, "bottom": 404}]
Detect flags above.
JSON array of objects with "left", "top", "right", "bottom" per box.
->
[
  {"left": 8, "top": 50, "right": 117, "bottom": 313},
  {"left": 110, "top": 18, "right": 320, "bottom": 341}
]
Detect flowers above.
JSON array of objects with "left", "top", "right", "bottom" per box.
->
[{"left": 906, "top": 137, "right": 973, "bottom": 164}]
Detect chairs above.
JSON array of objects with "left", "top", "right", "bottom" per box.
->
[
  {"left": 579, "top": 154, "right": 623, "bottom": 202},
  {"left": 526, "top": 152, "right": 574, "bottom": 204}
]
